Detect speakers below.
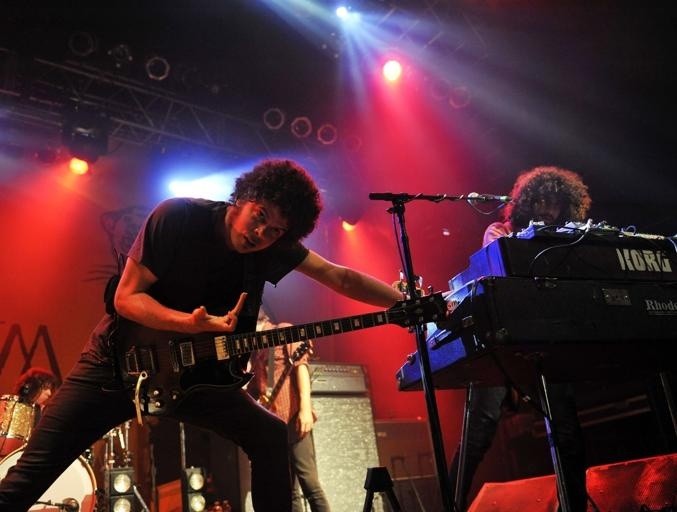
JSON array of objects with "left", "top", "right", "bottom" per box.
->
[
  {"left": 182, "top": 468, "right": 208, "bottom": 512},
  {"left": 464, "top": 472, "right": 561, "bottom": 512},
  {"left": 300, "top": 393, "right": 384, "bottom": 512},
  {"left": 584, "top": 452, "right": 677, "bottom": 512},
  {"left": 104, "top": 468, "right": 134, "bottom": 512}
]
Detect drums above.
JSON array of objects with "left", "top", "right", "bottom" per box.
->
[
  {"left": 1, "top": 442, "right": 99, "bottom": 511},
  {"left": 1, "top": 394, "right": 39, "bottom": 459}
]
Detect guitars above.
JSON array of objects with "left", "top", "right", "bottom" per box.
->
[
  {"left": 115, "top": 288, "right": 445, "bottom": 414},
  {"left": 255, "top": 340, "right": 314, "bottom": 412}
]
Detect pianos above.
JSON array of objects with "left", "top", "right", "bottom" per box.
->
[{"left": 394, "top": 231, "right": 677, "bottom": 391}]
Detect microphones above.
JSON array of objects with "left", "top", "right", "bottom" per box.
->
[{"left": 467, "top": 192, "right": 513, "bottom": 205}]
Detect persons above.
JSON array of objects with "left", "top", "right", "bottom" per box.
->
[
  {"left": 12, "top": 368, "right": 57, "bottom": 425},
  {"left": 246, "top": 308, "right": 330, "bottom": 512},
  {"left": 0, "top": 161, "right": 410, "bottom": 512},
  {"left": 449, "top": 167, "right": 593, "bottom": 512}
]
